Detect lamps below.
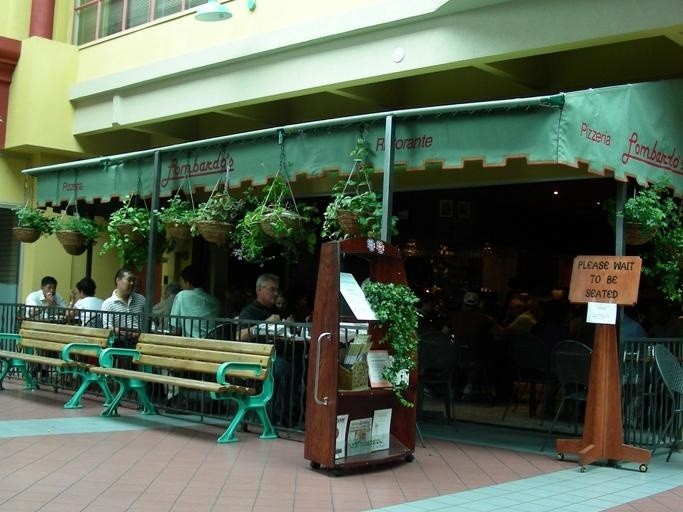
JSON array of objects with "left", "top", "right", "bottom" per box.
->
[{"left": 195, "top": 0, "right": 256, "bottom": 21}]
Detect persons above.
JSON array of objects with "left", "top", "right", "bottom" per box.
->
[
  {"left": 157, "top": 266, "right": 222, "bottom": 407},
  {"left": 276, "top": 294, "right": 285, "bottom": 308},
  {"left": 152, "top": 284, "right": 177, "bottom": 333},
  {"left": 235, "top": 273, "right": 293, "bottom": 341},
  {"left": 102, "top": 265, "right": 149, "bottom": 365},
  {"left": 509, "top": 296, "right": 538, "bottom": 329},
  {"left": 443, "top": 290, "right": 498, "bottom": 336},
  {"left": 24, "top": 276, "right": 69, "bottom": 321},
  {"left": 66, "top": 278, "right": 103, "bottom": 328}
]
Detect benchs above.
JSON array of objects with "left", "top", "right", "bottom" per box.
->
[
  {"left": 91, "top": 333, "right": 276, "bottom": 442},
  {"left": 0, "top": 320, "right": 115, "bottom": 409}
]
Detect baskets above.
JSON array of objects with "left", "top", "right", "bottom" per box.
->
[
  {"left": 336, "top": 209, "right": 366, "bottom": 233},
  {"left": 165, "top": 221, "right": 188, "bottom": 240},
  {"left": 196, "top": 221, "right": 235, "bottom": 243},
  {"left": 56, "top": 232, "right": 89, "bottom": 256},
  {"left": 118, "top": 223, "right": 144, "bottom": 245},
  {"left": 13, "top": 227, "right": 39, "bottom": 243},
  {"left": 260, "top": 214, "right": 298, "bottom": 239}
]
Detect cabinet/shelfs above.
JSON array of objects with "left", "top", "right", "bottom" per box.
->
[{"left": 304, "top": 241, "right": 416, "bottom": 475}]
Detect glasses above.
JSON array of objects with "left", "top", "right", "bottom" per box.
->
[{"left": 263, "top": 286, "right": 280, "bottom": 294}]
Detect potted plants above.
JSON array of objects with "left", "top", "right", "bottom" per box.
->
[
  {"left": 323, "top": 141, "right": 396, "bottom": 242},
  {"left": 162, "top": 169, "right": 198, "bottom": 240},
  {"left": 12, "top": 194, "right": 54, "bottom": 242},
  {"left": 193, "top": 167, "right": 241, "bottom": 246},
  {"left": 363, "top": 280, "right": 417, "bottom": 408},
  {"left": 100, "top": 179, "right": 156, "bottom": 266},
  {"left": 51, "top": 194, "right": 98, "bottom": 254},
  {"left": 607, "top": 175, "right": 683, "bottom": 302},
  {"left": 231, "top": 164, "right": 317, "bottom": 264}
]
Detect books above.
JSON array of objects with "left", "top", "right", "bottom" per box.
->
[
  {"left": 334, "top": 414, "right": 348, "bottom": 462},
  {"left": 372, "top": 409, "right": 393, "bottom": 452},
  {"left": 365, "top": 349, "right": 395, "bottom": 389},
  {"left": 348, "top": 420, "right": 375, "bottom": 458}
]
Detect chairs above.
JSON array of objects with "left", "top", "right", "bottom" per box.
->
[
  {"left": 502, "top": 335, "right": 561, "bottom": 426},
  {"left": 650, "top": 343, "right": 683, "bottom": 462},
  {"left": 166, "top": 323, "right": 240, "bottom": 414},
  {"left": 417, "top": 333, "right": 456, "bottom": 425},
  {"left": 540, "top": 340, "right": 593, "bottom": 453}
]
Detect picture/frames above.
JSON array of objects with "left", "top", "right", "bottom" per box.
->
[
  {"left": 456, "top": 199, "right": 472, "bottom": 219},
  {"left": 439, "top": 198, "right": 454, "bottom": 218}
]
